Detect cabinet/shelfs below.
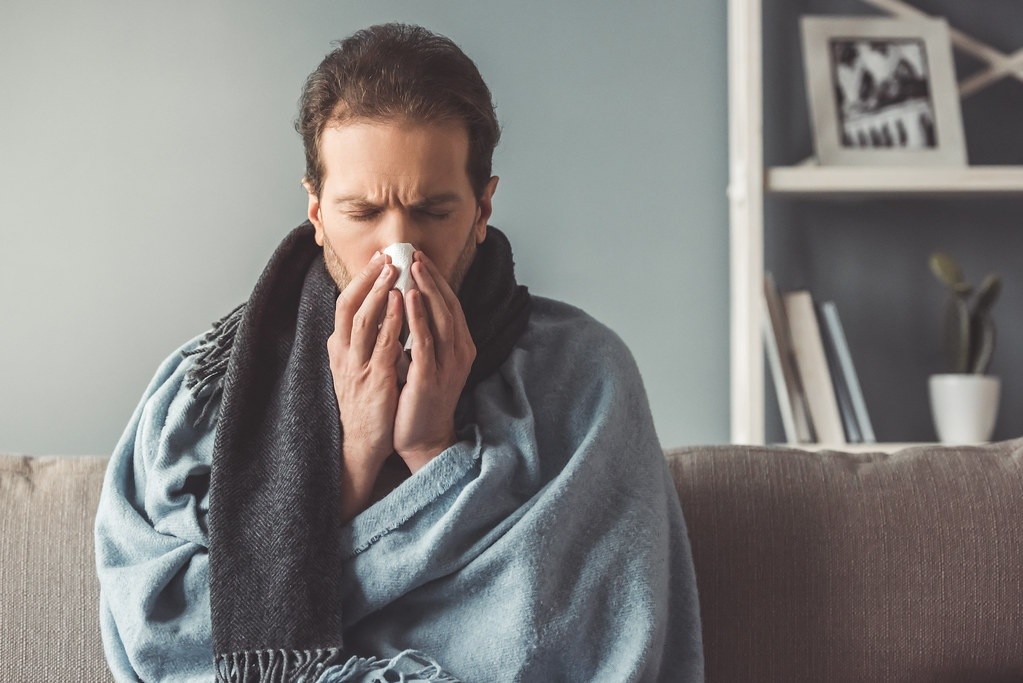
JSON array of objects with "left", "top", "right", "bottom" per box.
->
[{"left": 727, "top": 0, "right": 1022, "bottom": 454}]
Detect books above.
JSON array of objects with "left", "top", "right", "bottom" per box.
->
[{"left": 762, "top": 272, "right": 879, "bottom": 444}]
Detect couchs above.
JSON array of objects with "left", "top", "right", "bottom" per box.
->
[{"left": 0, "top": 446, "right": 1022, "bottom": 683}]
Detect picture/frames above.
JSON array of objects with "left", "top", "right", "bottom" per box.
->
[{"left": 798, "top": 13, "right": 971, "bottom": 166}]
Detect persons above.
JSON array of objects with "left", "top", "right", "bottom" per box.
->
[{"left": 95, "top": 27, "right": 706, "bottom": 683}]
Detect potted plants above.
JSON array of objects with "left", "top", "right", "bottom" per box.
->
[{"left": 921, "top": 251, "right": 1003, "bottom": 445}]
